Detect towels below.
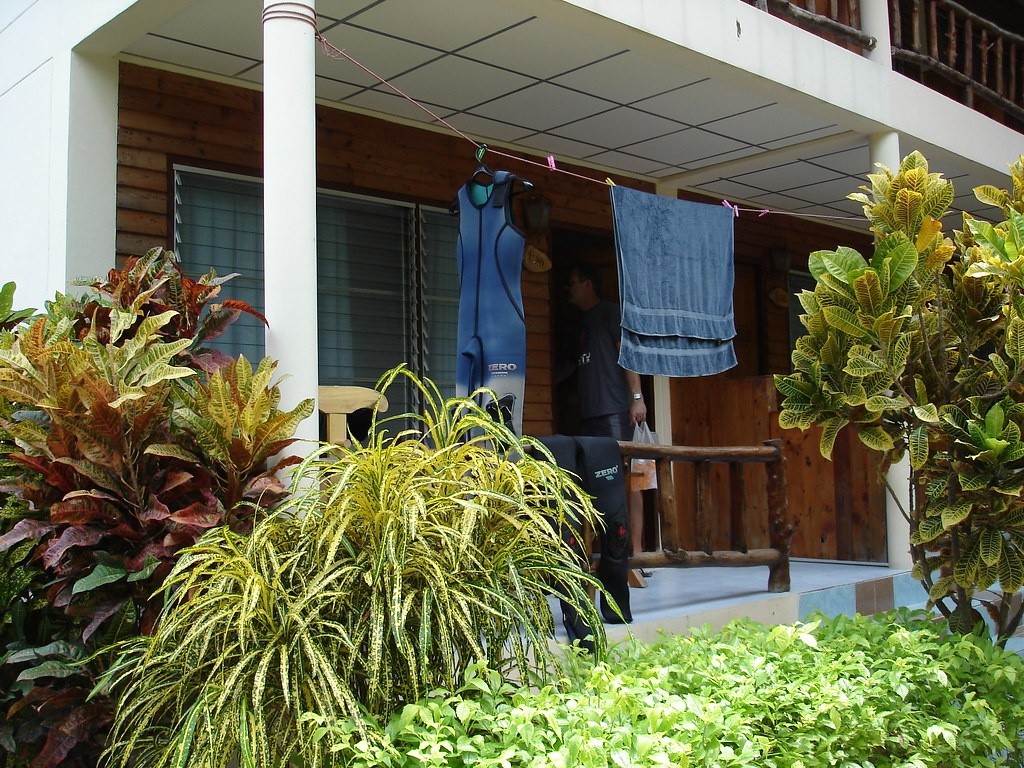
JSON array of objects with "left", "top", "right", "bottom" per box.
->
[{"left": 608, "top": 184, "right": 738, "bottom": 378}]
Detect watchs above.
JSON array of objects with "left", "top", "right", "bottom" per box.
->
[{"left": 631, "top": 391, "right": 644, "bottom": 401}]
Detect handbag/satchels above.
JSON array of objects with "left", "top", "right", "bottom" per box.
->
[{"left": 630, "top": 419, "right": 659, "bottom": 492}]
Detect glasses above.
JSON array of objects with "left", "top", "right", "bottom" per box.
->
[{"left": 564, "top": 279, "right": 575, "bottom": 288}]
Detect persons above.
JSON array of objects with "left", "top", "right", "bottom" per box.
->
[{"left": 551, "top": 267, "right": 656, "bottom": 577}]
